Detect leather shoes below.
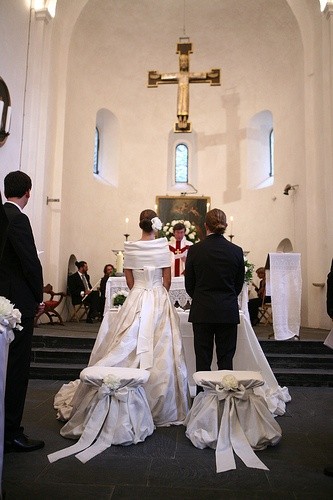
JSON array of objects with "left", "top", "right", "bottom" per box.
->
[{"left": 3, "top": 433, "right": 45, "bottom": 453}]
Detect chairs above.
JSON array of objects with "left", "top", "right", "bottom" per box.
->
[
  {"left": 65, "top": 287, "right": 91, "bottom": 325},
  {"left": 34, "top": 283, "right": 67, "bottom": 327},
  {"left": 257, "top": 294, "right": 274, "bottom": 326}
]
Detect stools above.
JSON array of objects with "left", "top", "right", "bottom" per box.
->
[
  {"left": 73, "top": 369, "right": 153, "bottom": 445},
  {"left": 191, "top": 369, "right": 264, "bottom": 453}
]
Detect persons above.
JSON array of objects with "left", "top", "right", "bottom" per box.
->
[
  {"left": 323, "top": 260, "right": 333, "bottom": 352},
  {"left": 149, "top": 55, "right": 218, "bottom": 129},
  {"left": 247, "top": 267, "right": 272, "bottom": 326},
  {"left": 0, "top": 170, "right": 45, "bottom": 455},
  {"left": 90, "top": 209, "right": 190, "bottom": 427},
  {"left": 66, "top": 261, "right": 119, "bottom": 326},
  {"left": 184, "top": 210, "right": 245, "bottom": 395},
  {"left": 167, "top": 223, "right": 194, "bottom": 276}
]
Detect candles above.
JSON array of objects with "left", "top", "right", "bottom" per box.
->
[
  {"left": 230, "top": 215, "right": 233, "bottom": 233},
  {"left": 115, "top": 250, "right": 123, "bottom": 273},
  {"left": 124, "top": 216, "right": 129, "bottom": 233}
]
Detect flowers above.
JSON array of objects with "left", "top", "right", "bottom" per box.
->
[
  {"left": 111, "top": 289, "right": 130, "bottom": 308},
  {"left": 162, "top": 218, "right": 202, "bottom": 243}
]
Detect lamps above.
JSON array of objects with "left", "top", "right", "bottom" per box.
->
[
  {"left": 33, "top": 0, "right": 58, "bottom": 23},
  {"left": 283, "top": 182, "right": 297, "bottom": 196}
]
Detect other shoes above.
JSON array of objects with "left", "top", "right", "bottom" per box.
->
[{"left": 86, "top": 318, "right": 97, "bottom": 322}]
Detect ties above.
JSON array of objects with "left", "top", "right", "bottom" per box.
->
[{"left": 81, "top": 275, "right": 87, "bottom": 290}]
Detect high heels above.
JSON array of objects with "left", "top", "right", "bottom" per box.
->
[{"left": 252, "top": 319, "right": 260, "bottom": 327}]
